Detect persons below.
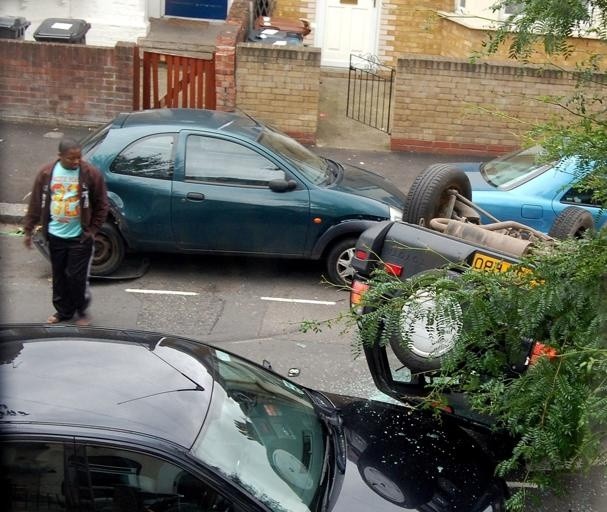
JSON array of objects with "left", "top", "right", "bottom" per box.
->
[{"left": 22, "top": 140, "right": 108, "bottom": 326}]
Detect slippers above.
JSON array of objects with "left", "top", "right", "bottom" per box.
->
[{"left": 48, "top": 312, "right": 73, "bottom": 324}]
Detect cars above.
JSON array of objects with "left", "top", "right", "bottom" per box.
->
[
  {"left": 1, "top": 321, "right": 532, "bottom": 511},
  {"left": 440, "top": 145, "right": 606, "bottom": 241},
  {"left": 70, "top": 109, "right": 413, "bottom": 288}
]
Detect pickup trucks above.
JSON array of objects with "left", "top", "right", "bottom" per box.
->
[{"left": 348, "top": 219, "right": 571, "bottom": 409}]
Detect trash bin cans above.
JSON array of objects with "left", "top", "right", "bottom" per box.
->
[
  {"left": 33, "top": 18, "right": 91, "bottom": 45},
  {"left": 0, "top": 15, "right": 31, "bottom": 40},
  {"left": 245, "top": 15, "right": 311, "bottom": 48}
]
